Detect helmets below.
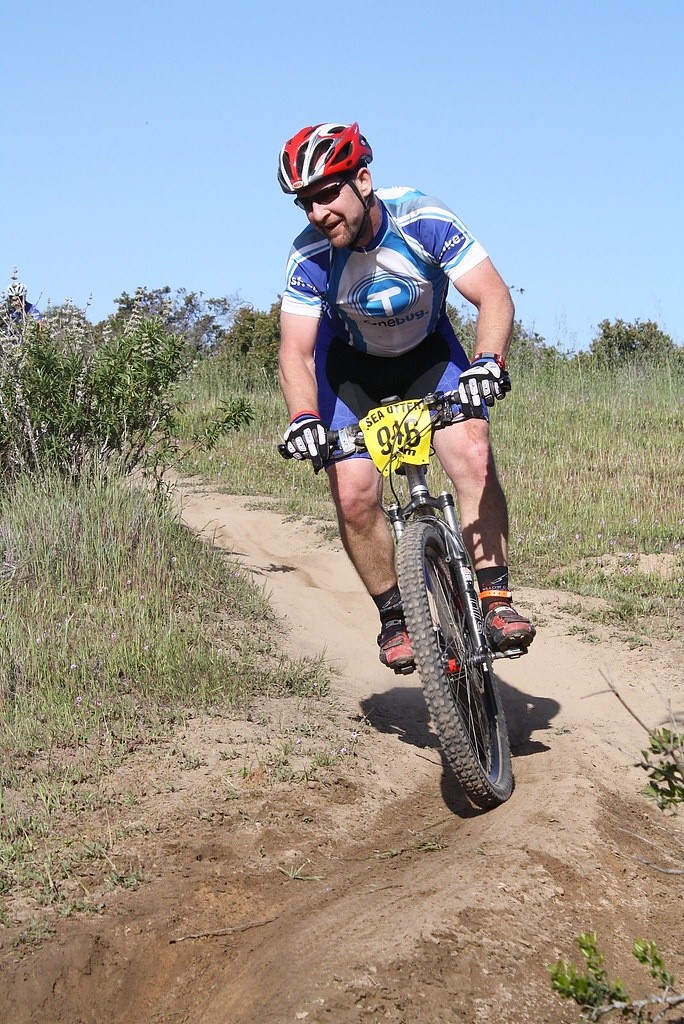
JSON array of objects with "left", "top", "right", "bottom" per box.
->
[{"left": 277, "top": 123, "right": 374, "bottom": 194}]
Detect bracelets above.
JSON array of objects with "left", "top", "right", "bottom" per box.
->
[
  {"left": 289, "top": 410, "right": 321, "bottom": 423},
  {"left": 471, "top": 352, "right": 507, "bottom": 369}
]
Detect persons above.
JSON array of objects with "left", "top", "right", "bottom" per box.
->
[{"left": 277, "top": 125, "right": 538, "bottom": 668}]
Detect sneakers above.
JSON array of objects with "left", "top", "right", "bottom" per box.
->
[
  {"left": 379, "top": 616, "right": 415, "bottom": 665},
  {"left": 484, "top": 602, "right": 536, "bottom": 651}
]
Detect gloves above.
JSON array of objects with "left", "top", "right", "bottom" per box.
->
[
  {"left": 283, "top": 411, "right": 328, "bottom": 467},
  {"left": 458, "top": 351, "right": 507, "bottom": 416}
]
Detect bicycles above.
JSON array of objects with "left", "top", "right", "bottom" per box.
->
[{"left": 277, "top": 373, "right": 530, "bottom": 810}]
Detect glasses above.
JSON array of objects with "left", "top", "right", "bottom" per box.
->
[{"left": 294, "top": 174, "right": 355, "bottom": 210}]
